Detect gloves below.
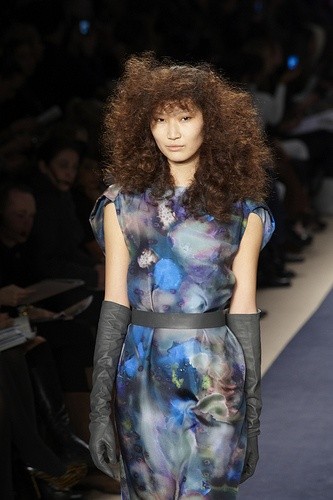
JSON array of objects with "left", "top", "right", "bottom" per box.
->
[
  {"left": 226, "top": 306, "right": 263, "bottom": 481},
  {"left": 87, "top": 301, "right": 132, "bottom": 478}
]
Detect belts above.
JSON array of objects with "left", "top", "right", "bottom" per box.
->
[{"left": 130, "top": 309, "right": 228, "bottom": 330}]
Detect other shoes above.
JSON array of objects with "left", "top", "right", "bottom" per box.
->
[{"left": 266, "top": 233, "right": 314, "bottom": 288}]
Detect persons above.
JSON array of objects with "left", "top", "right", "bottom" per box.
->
[
  {"left": 0, "top": 0, "right": 333, "bottom": 500},
  {"left": 87, "top": 55, "right": 276, "bottom": 500}
]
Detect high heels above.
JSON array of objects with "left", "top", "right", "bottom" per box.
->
[{"left": 25, "top": 457, "right": 87, "bottom": 500}]
